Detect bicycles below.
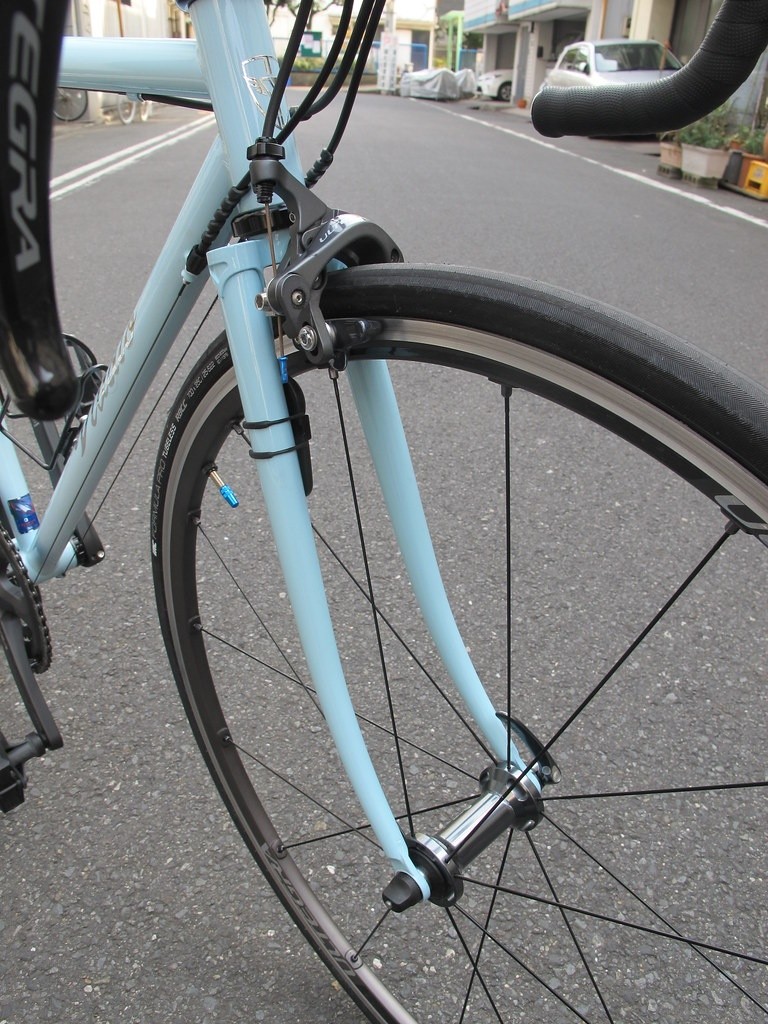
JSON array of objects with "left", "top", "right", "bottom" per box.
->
[
  {"left": 54, "top": 86, "right": 89, "bottom": 122},
  {"left": 1, "top": 2, "right": 766, "bottom": 1022},
  {"left": 116, "top": 94, "right": 152, "bottom": 125}
]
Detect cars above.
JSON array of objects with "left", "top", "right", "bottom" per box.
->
[
  {"left": 540, "top": 38, "right": 685, "bottom": 86},
  {"left": 475, "top": 70, "right": 513, "bottom": 100}
]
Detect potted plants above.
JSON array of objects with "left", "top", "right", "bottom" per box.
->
[
  {"left": 462, "top": 32, "right": 483, "bottom": 50},
  {"left": 658, "top": 98, "right": 768, "bottom": 188}
]
